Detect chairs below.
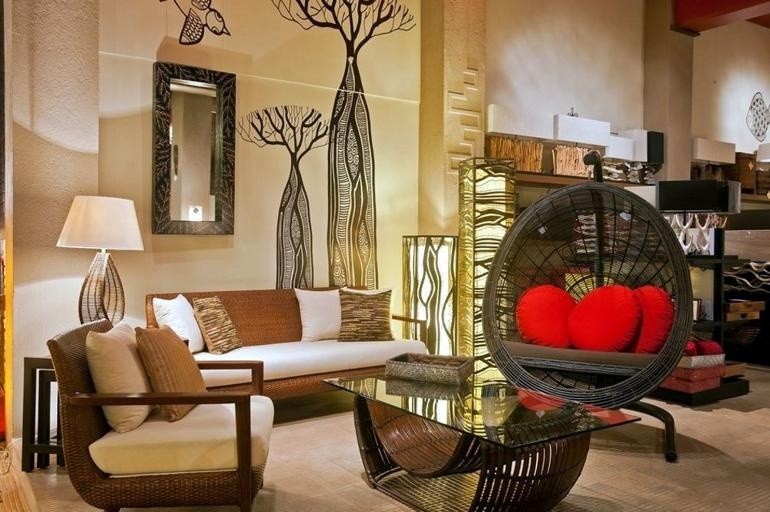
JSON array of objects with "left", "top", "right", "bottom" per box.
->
[{"left": 46, "top": 318, "right": 276, "bottom": 512}]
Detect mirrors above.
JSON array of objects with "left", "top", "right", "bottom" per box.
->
[{"left": 151, "top": 61, "right": 236, "bottom": 236}]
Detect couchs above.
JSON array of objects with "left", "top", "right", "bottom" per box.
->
[
  {"left": 147, "top": 286, "right": 428, "bottom": 402},
  {"left": 481, "top": 181, "right": 694, "bottom": 464}
]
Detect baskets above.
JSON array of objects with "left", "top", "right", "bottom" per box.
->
[{"left": 385, "top": 352, "right": 475, "bottom": 386}]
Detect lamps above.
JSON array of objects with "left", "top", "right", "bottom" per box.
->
[
  {"left": 402, "top": 234, "right": 459, "bottom": 357},
  {"left": 57, "top": 195, "right": 145, "bottom": 326}
]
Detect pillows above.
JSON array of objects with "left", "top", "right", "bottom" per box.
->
[
  {"left": 515, "top": 284, "right": 674, "bottom": 355},
  {"left": 85, "top": 318, "right": 206, "bottom": 434},
  {"left": 151, "top": 293, "right": 243, "bottom": 355},
  {"left": 294, "top": 287, "right": 396, "bottom": 343}
]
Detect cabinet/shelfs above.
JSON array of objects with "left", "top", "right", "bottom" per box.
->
[{"left": 484, "top": 134, "right": 606, "bottom": 287}]
